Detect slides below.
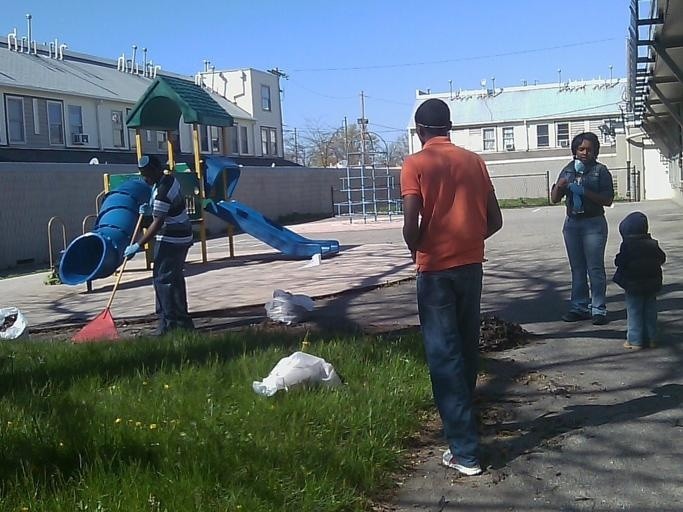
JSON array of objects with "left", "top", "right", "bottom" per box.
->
[
  {"left": 205, "top": 198, "right": 339, "bottom": 257},
  {"left": 59, "top": 181, "right": 152, "bottom": 285}
]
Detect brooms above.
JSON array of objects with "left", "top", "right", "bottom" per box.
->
[{"left": 69, "top": 213, "right": 145, "bottom": 342}]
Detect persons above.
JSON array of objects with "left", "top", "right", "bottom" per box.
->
[
  {"left": 397, "top": 98, "right": 503, "bottom": 476},
  {"left": 122, "top": 155, "right": 195, "bottom": 334},
  {"left": 548, "top": 132, "right": 615, "bottom": 326},
  {"left": 611, "top": 211, "right": 666, "bottom": 351}
]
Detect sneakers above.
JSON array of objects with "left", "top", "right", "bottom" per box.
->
[
  {"left": 623, "top": 341, "right": 656, "bottom": 349},
  {"left": 562, "top": 309, "right": 608, "bottom": 325},
  {"left": 442, "top": 450, "right": 483, "bottom": 476}
]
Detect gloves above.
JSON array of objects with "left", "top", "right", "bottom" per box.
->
[
  {"left": 123, "top": 242, "right": 140, "bottom": 260},
  {"left": 138, "top": 203, "right": 152, "bottom": 216}
]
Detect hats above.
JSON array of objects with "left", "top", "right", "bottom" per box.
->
[{"left": 415, "top": 98, "right": 452, "bottom": 129}]
